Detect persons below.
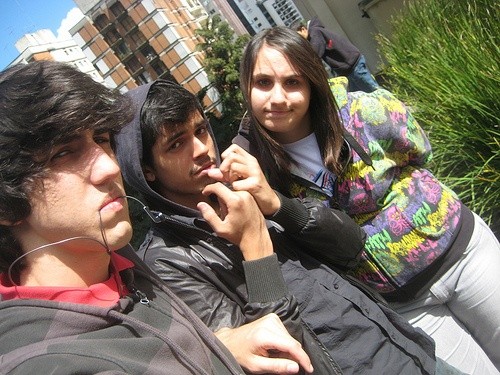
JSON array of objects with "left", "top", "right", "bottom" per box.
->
[
  {"left": 0, "top": 62, "right": 314, "bottom": 374},
  {"left": 233, "top": 27, "right": 500, "bottom": 374},
  {"left": 111, "top": 79, "right": 467, "bottom": 374},
  {"left": 292, "top": 15, "right": 384, "bottom": 93}
]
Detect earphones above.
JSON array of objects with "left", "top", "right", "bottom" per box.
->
[{"left": 143, "top": 206, "right": 165, "bottom": 223}]
